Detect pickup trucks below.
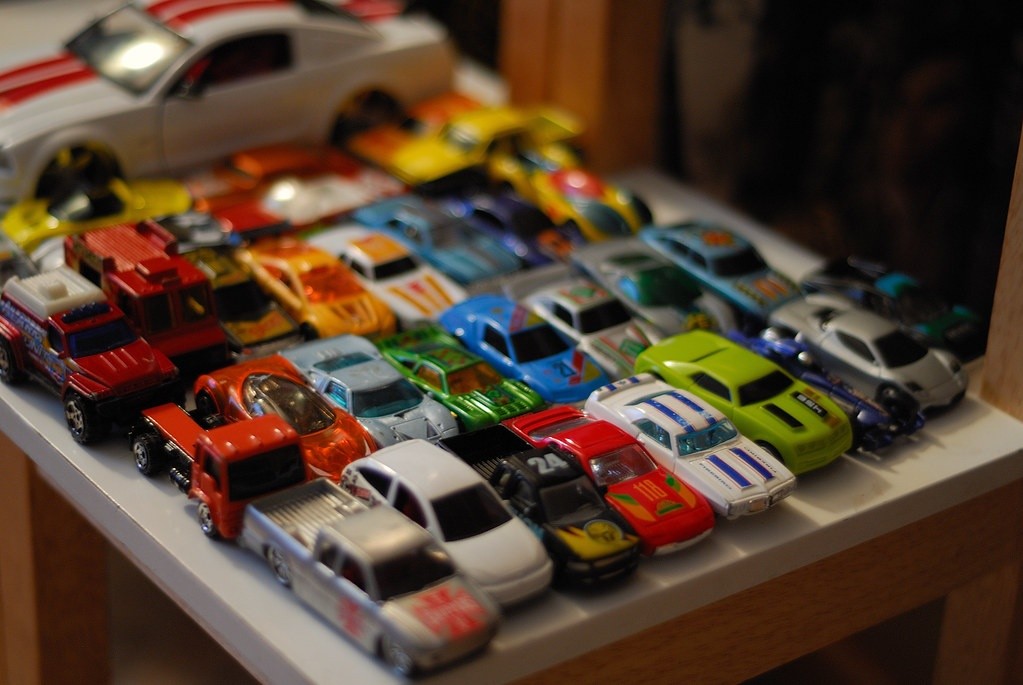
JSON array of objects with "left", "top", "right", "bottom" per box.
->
[
  {"left": 239, "top": 473, "right": 501, "bottom": 680},
  {"left": 436, "top": 422, "right": 646, "bottom": 593}
]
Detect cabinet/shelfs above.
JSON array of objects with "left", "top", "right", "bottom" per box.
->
[{"left": 0, "top": 0, "right": 1023, "bottom": 685}]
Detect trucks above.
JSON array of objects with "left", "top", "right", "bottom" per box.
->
[
  {"left": 0, "top": 265, "right": 182, "bottom": 447},
  {"left": 63, "top": 220, "right": 242, "bottom": 383},
  {"left": 125, "top": 401, "right": 311, "bottom": 545}
]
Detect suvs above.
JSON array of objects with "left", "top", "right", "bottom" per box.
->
[{"left": 337, "top": 438, "right": 557, "bottom": 609}]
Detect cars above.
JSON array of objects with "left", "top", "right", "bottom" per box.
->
[
  {"left": 0, "top": 65, "right": 802, "bottom": 338},
  {"left": 435, "top": 289, "right": 612, "bottom": 405},
  {"left": 583, "top": 370, "right": 799, "bottom": 520},
  {"left": 190, "top": 352, "right": 379, "bottom": 484},
  {"left": 284, "top": 331, "right": 459, "bottom": 450},
  {"left": 632, "top": 327, "right": 855, "bottom": 476},
  {"left": 799, "top": 246, "right": 985, "bottom": 347},
  {"left": 767, "top": 290, "right": 969, "bottom": 420},
  {"left": 502, "top": 404, "right": 716, "bottom": 557},
  {"left": 372, "top": 319, "right": 543, "bottom": 432},
  {"left": 725, "top": 326, "right": 921, "bottom": 458},
  {"left": 0, "top": 0, "right": 458, "bottom": 205}
]
